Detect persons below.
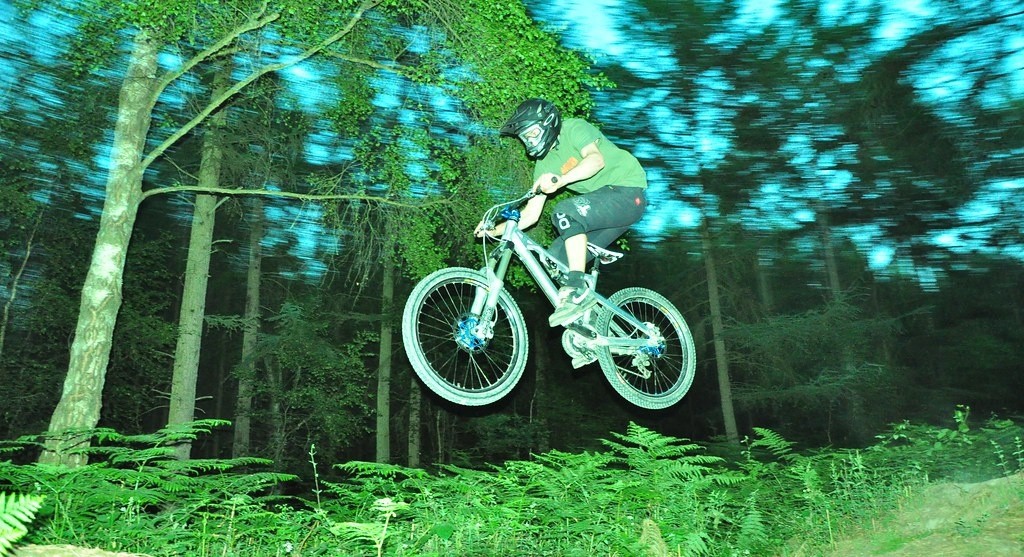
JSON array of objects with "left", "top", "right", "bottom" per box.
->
[{"left": 472, "top": 98, "right": 648, "bottom": 328}]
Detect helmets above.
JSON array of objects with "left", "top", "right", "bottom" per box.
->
[{"left": 500, "top": 98, "right": 561, "bottom": 160}]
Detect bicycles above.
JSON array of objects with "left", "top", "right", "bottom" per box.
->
[{"left": 399, "top": 171, "right": 699, "bottom": 410}]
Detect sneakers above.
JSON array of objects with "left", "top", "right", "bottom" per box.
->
[
  {"left": 572, "top": 334, "right": 616, "bottom": 369},
  {"left": 549, "top": 282, "right": 599, "bottom": 328}
]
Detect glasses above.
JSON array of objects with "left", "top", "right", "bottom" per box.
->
[{"left": 518, "top": 107, "right": 558, "bottom": 149}]
